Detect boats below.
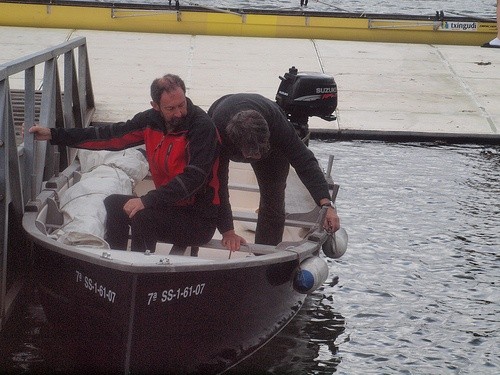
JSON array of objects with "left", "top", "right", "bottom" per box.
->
[{"left": 21, "top": 65, "right": 347, "bottom": 375}]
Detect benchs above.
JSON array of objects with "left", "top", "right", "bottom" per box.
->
[{"left": 166, "top": 183, "right": 318, "bottom": 253}]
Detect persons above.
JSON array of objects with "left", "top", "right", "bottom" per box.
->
[
  {"left": 204, "top": 92, "right": 340, "bottom": 254},
  {"left": 22, "top": 75, "right": 227, "bottom": 256},
  {"left": 482, "top": 0, "right": 500, "bottom": 47}
]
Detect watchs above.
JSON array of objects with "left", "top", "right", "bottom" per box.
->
[{"left": 322, "top": 201, "right": 336, "bottom": 211}]
